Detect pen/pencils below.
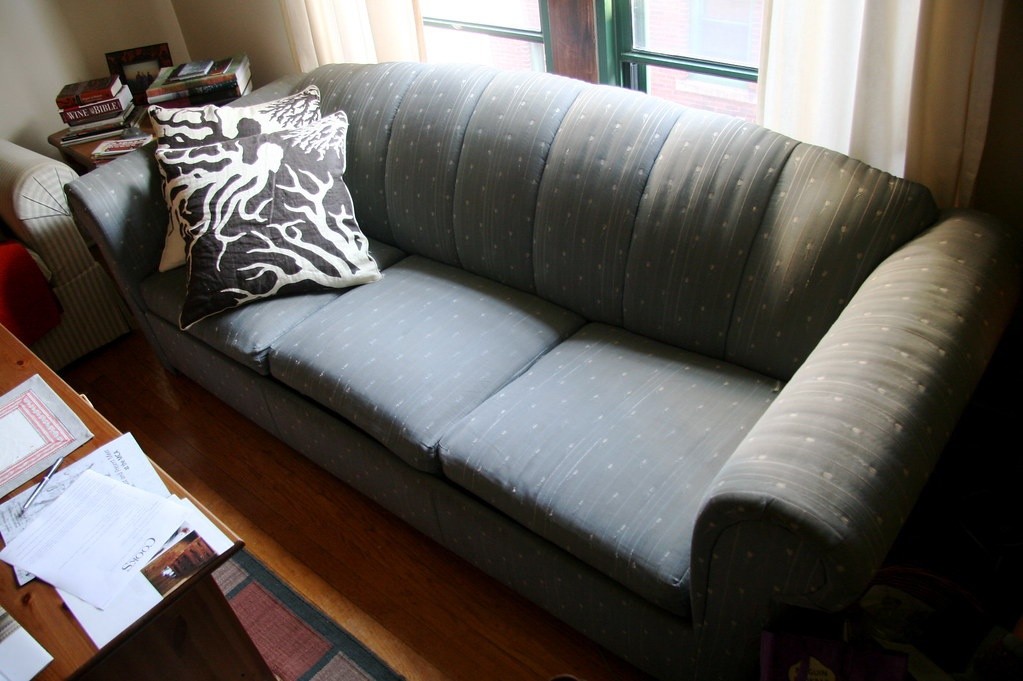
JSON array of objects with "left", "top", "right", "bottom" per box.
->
[{"left": 18, "top": 456, "right": 64, "bottom": 517}]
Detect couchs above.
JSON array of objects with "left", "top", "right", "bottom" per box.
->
[
  {"left": 0, "top": 137, "right": 129, "bottom": 371},
  {"left": 59, "top": 59, "right": 1023, "bottom": 681}
]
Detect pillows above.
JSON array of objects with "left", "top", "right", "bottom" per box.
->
[
  {"left": 154, "top": 111, "right": 382, "bottom": 331},
  {"left": 148, "top": 84, "right": 322, "bottom": 274}
]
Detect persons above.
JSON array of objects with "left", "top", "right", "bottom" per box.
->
[{"left": 135, "top": 71, "right": 154, "bottom": 94}]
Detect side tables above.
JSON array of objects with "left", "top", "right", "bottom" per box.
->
[{"left": 47, "top": 107, "right": 155, "bottom": 172}]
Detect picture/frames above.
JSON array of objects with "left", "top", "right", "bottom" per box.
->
[{"left": 105, "top": 41, "right": 174, "bottom": 103}]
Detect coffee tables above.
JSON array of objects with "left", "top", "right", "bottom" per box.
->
[{"left": 0, "top": 322, "right": 274, "bottom": 681}]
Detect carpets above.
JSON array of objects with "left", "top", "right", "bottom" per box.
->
[{"left": 213, "top": 545, "right": 410, "bottom": 681}]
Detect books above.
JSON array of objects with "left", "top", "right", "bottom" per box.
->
[
  {"left": 146, "top": 53, "right": 253, "bottom": 111},
  {"left": 55, "top": 74, "right": 147, "bottom": 147},
  {"left": 90, "top": 135, "right": 153, "bottom": 163}
]
